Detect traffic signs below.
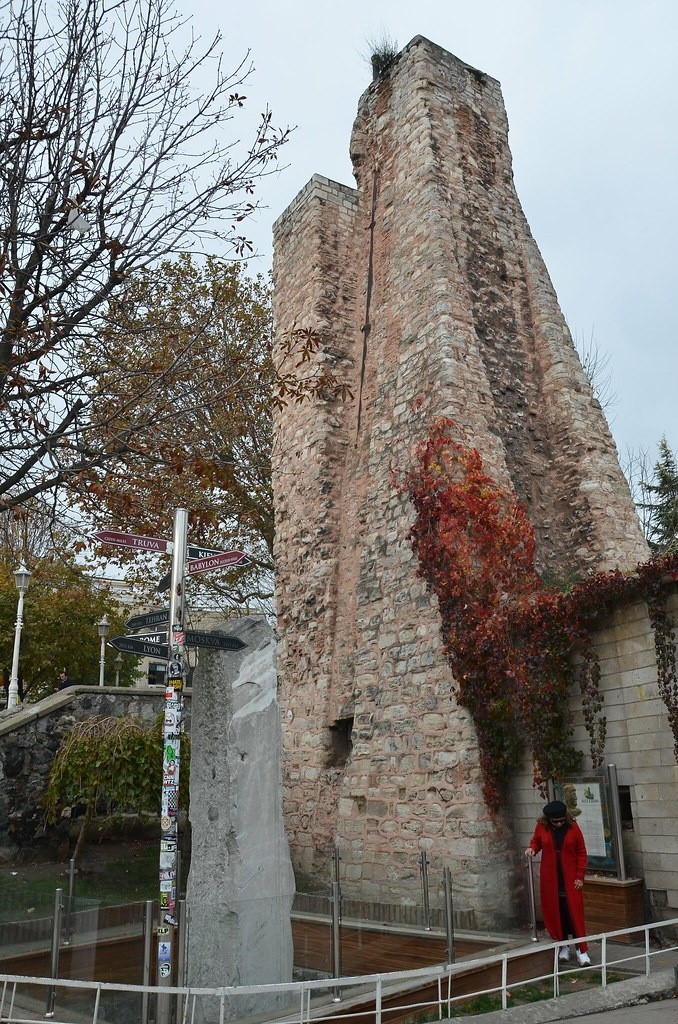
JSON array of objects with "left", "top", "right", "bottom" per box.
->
[{"left": 91, "top": 531, "right": 252, "bottom": 660}]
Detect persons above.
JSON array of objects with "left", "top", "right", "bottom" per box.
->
[{"left": 523, "top": 799, "right": 593, "bottom": 968}]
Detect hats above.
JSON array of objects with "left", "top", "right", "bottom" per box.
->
[{"left": 543, "top": 800, "right": 568, "bottom": 816}]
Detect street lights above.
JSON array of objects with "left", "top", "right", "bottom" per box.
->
[
  {"left": 8, "top": 558, "right": 33, "bottom": 709},
  {"left": 114, "top": 653, "right": 124, "bottom": 686},
  {"left": 98, "top": 615, "right": 110, "bottom": 686}
]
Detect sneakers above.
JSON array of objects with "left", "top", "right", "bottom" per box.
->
[
  {"left": 558, "top": 944, "right": 571, "bottom": 962},
  {"left": 575, "top": 949, "right": 591, "bottom": 967}
]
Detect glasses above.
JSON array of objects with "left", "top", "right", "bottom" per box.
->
[{"left": 551, "top": 817, "right": 567, "bottom": 823}]
